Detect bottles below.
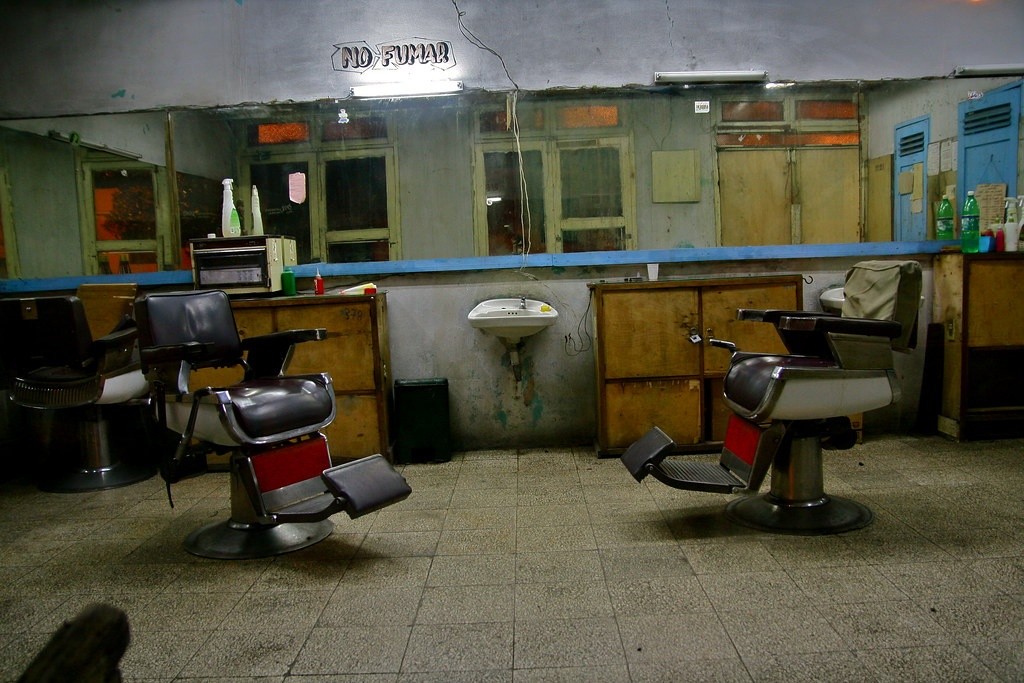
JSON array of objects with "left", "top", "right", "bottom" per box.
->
[
  {"left": 314, "top": 267, "right": 324, "bottom": 295},
  {"left": 936, "top": 195, "right": 954, "bottom": 240},
  {"left": 281, "top": 268, "right": 296, "bottom": 296},
  {"left": 961, "top": 190, "right": 980, "bottom": 254},
  {"left": 250, "top": 185, "right": 265, "bottom": 237},
  {"left": 995, "top": 227, "right": 1004, "bottom": 252}
]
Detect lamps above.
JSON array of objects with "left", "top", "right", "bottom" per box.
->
[
  {"left": 653, "top": 69, "right": 770, "bottom": 84},
  {"left": 952, "top": 64, "right": 1024, "bottom": 79}
]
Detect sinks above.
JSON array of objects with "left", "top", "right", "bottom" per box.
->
[{"left": 467, "top": 298, "right": 559, "bottom": 337}]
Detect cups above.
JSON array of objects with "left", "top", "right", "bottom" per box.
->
[{"left": 647, "top": 264, "right": 659, "bottom": 282}]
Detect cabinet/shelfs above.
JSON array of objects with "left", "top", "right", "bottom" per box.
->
[
  {"left": 165, "top": 291, "right": 393, "bottom": 465},
  {"left": 586, "top": 274, "right": 803, "bottom": 459}
]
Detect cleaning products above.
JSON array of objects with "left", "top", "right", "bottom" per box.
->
[
  {"left": 251, "top": 185, "right": 264, "bottom": 235},
  {"left": 222, "top": 179, "right": 241, "bottom": 238}
]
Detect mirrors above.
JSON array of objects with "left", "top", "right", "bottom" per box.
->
[{"left": 0, "top": 71, "right": 1024, "bottom": 297}]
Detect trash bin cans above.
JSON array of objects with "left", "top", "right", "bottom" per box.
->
[{"left": 394, "top": 378, "right": 453, "bottom": 465}]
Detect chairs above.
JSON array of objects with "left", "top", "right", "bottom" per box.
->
[
  {"left": 0, "top": 294, "right": 163, "bottom": 493},
  {"left": 621, "top": 260, "right": 922, "bottom": 537},
  {"left": 133, "top": 287, "right": 413, "bottom": 562}
]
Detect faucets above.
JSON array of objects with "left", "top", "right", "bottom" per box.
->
[{"left": 519, "top": 296, "right": 527, "bottom": 309}]
